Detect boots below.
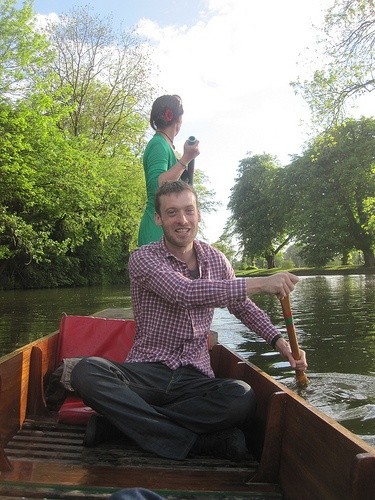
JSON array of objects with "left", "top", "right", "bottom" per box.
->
[
  {"left": 187, "top": 426, "right": 247, "bottom": 461},
  {"left": 83, "top": 414, "right": 136, "bottom": 449}
]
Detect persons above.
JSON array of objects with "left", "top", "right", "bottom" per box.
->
[
  {"left": 70, "top": 180, "right": 308, "bottom": 464},
  {"left": 137, "top": 94, "right": 201, "bottom": 247}
]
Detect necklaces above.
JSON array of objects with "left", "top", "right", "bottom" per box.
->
[{"left": 156, "top": 128, "right": 176, "bottom": 150}]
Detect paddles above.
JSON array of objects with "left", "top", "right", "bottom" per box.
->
[
  {"left": 277, "top": 290, "right": 308, "bottom": 384},
  {"left": 189, "top": 134, "right": 197, "bottom": 186}
]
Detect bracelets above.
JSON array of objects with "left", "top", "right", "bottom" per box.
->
[
  {"left": 271, "top": 332, "right": 282, "bottom": 350},
  {"left": 178, "top": 159, "right": 188, "bottom": 170}
]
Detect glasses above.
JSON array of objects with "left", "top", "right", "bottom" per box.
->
[{"left": 173, "top": 94, "right": 183, "bottom": 111}]
